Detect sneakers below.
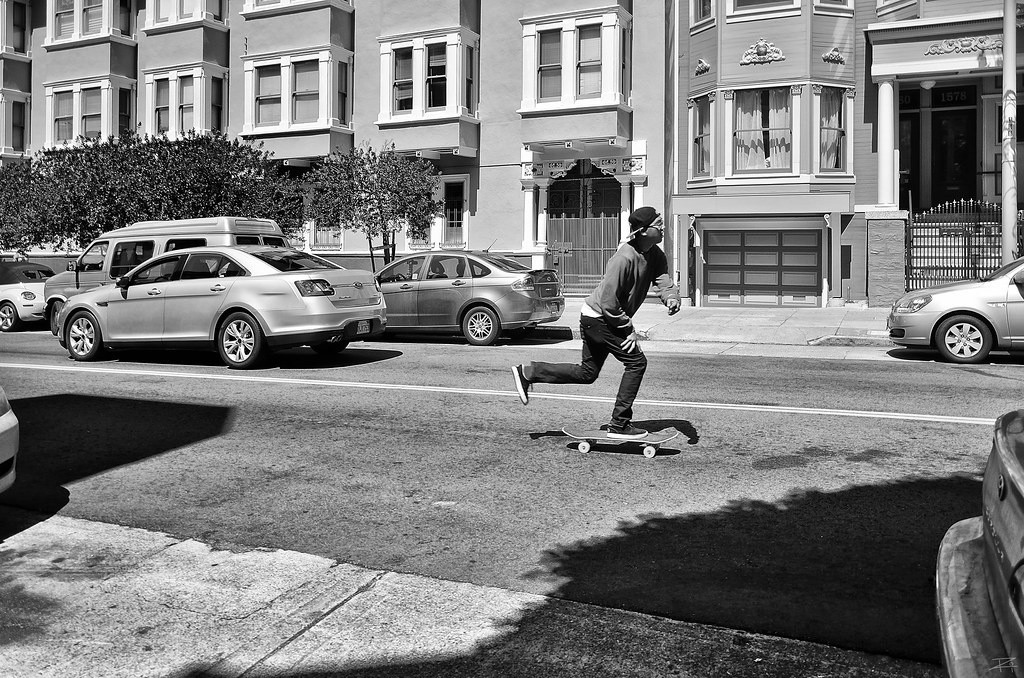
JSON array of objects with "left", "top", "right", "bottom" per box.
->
[
  {"left": 607, "top": 422, "right": 649, "bottom": 439},
  {"left": 511, "top": 364, "right": 533, "bottom": 405}
]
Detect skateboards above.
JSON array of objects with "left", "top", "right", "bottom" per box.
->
[{"left": 562, "top": 425, "right": 679, "bottom": 458}]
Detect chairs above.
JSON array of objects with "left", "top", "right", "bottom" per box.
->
[
  {"left": 431, "top": 263, "right": 448, "bottom": 279},
  {"left": 187, "top": 264, "right": 214, "bottom": 279},
  {"left": 457, "top": 261, "right": 466, "bottom": 278},
  {"left": 118, "top": 250, "right": 139, "bottom": 277}
]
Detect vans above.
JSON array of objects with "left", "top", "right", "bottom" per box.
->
[{"left": 45, "top": 217, "right": 290, "bottom": 337}]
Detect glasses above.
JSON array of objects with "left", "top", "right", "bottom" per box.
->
[{"left": 648, "top": 225, "right": 665, "bottom": 231}]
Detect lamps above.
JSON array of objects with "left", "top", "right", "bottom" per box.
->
[{"left": 920, "top": 80, "right": 937, "bottom": 91}]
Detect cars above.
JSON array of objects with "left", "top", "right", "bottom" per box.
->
[
  {"left": 0, "top": 262, "right": 57, "bottom": 334},
  {"left": 58, "top": 246, "right": 387, "bottom": 370},
  {"left": 887, "top": 256, "right": 1024, "bottom": 364},
  {"left": 373, "top": 250, "right": 564, "bottom": 346}
]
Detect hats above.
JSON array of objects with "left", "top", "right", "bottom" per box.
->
[{"left": 625, "top": 207, "right": 661, "bottom": 238}]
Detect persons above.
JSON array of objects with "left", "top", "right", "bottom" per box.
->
[{"left": 512, "top": 207, "right": 681, "bottom": 439}]
